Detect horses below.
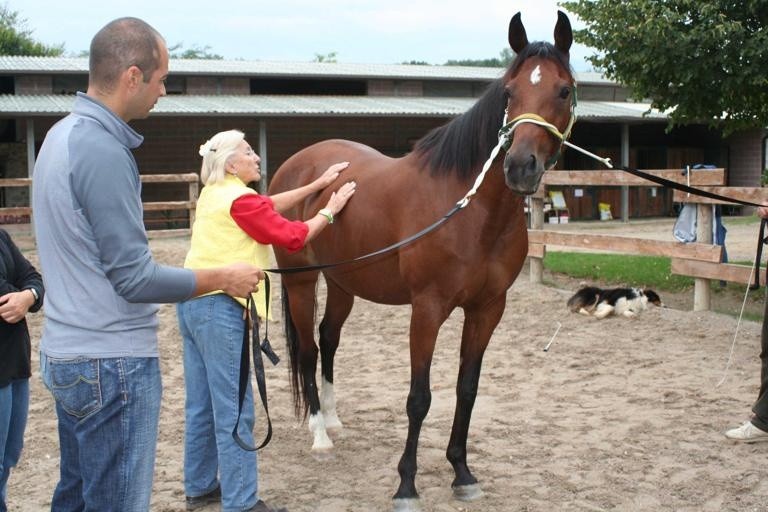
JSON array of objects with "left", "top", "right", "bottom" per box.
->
[{"left": 259, "top": 6, "right": 577, "bottom": 512}]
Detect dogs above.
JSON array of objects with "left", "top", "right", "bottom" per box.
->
[{"left": 565, "top": 284, "right": 667, "bottom": 323}]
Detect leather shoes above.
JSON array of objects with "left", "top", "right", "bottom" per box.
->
[
  {"left": 249, "top": 500, "right": 285, "bottom": 512},
  {"left": 185, "top": 484, "right": 221, "bottom": 509}
]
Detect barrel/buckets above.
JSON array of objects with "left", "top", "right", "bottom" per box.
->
[
  {"left": 560, "top": 215, "right": 568, "bottom": 223},
  {"left": 549, "top": 217, "right": 558, "bottom": 224}
]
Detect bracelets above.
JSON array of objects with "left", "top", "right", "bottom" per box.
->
[
  {"left": 318, "top": 208, "right": 334, "bottom": 224},
  {"left": 30, "top": 288, "right": 39, "bottom": 301}
]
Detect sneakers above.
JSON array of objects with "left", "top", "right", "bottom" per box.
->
[{"left": 725, "top": 421, "right": 768, "bottom": 441}]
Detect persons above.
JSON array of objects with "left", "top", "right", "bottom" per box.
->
[
  {"left": 30, "top": 13, "right": 266, "bottom": 512},
  {"left": 178, "top": 128, "right": 360, "bottom": 512},
  {"left": 725, "top": 196, "right": 768, "bottom": 443},
  {"left": 0, "top": 228, "right": 47, "bottom": 512}
]
